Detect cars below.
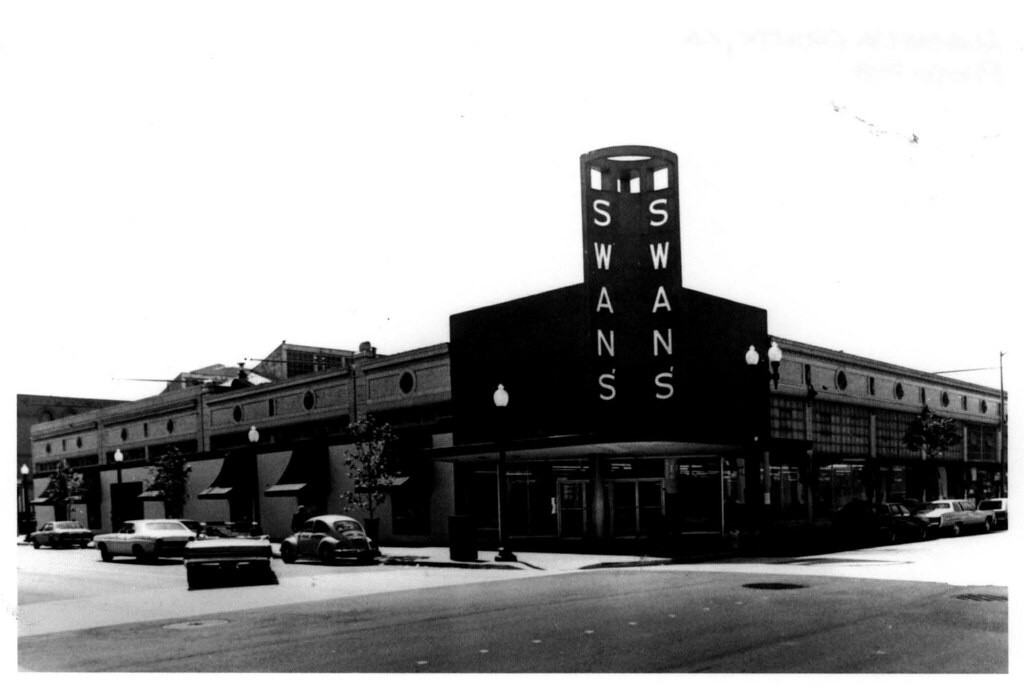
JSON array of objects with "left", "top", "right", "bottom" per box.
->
[
  {"left": 184, "top": 521, "right": 272, "bottom": 588},
  {"left": 914, "top": 499, "right": 995, "bottom": 537},
  {"left": 31, "top": 520, "right": 93, "bottom": 549},
  {"left": 280, "top": 514, "right": 378, "bottom": 564},
  {"left": 977, "top": 497, "right": 1008, "bottom": 530},
  {"left": 825, "top": 498, "right": 931, "bottom": 544},
  {"left": 93, "top": 519, "right": 197, "bottom": 565}
]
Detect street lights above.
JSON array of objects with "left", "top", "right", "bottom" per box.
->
[
  {"left": 493, "top": 384, "right": 517, "bottom": 561},
  {"left": 248, "top": 426, "right": 260, "bottom": 520},
  {"left": 20, "top": 463, "right": 31, "bottom": 542},
  {"left": 114, "top": 448, "right": 124, "bottom": 532}
]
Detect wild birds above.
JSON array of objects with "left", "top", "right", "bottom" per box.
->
[
  {"left": 910, "top": 133, "right": 919, "bottom": 144},
  {"left": 834, "top": 105, "right": 839, "bottom": 112}
]
